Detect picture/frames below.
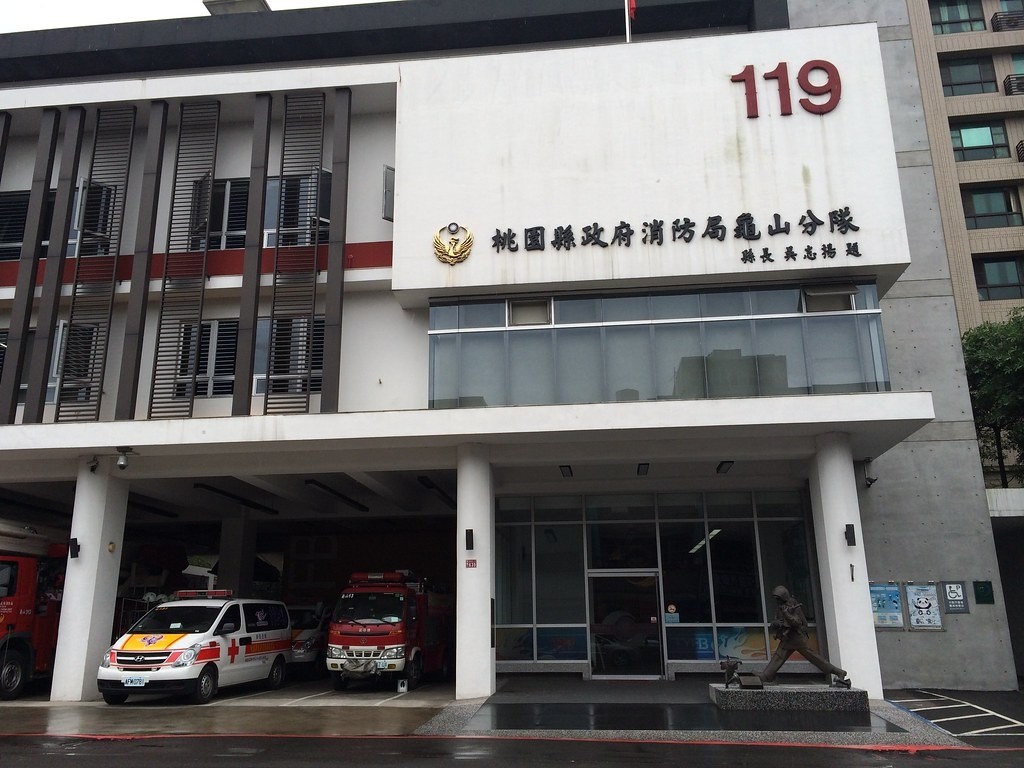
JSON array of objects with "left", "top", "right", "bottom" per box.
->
[
  {"left": 869, "top": 580, "right": 908, "bottom": 632},
  {"left": 902, "top": 580, "right": 947, "bottom": 632}
]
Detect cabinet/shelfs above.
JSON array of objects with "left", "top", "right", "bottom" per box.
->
[{"left": 112, "top": 584, "right": 164, "bottom": 644}]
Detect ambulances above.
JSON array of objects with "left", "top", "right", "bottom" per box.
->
[
  {"left": 287, "top": 604, "right": 326, "bottom": 674},
  {"left": 96, "top": 590, "right": 294, "bottom": 704}
]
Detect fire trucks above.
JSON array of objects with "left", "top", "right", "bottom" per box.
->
[
  {"left": 0, "top": 518, "right": 170, "bottom": 702},
  {"left": 324, "top": 571, "right": 440, "bottom": 695}
]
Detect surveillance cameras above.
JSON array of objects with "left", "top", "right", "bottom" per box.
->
[
  {"left": 865, "top": 476, "right": 879, "bottom": 488},
  {"left": 117, "top": 455, "right": 129, "bottom": 470},
  {"left": 87, "top": 456, "right": 99, "bottom": 467}
]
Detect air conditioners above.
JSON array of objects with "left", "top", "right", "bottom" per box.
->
[
  {"left": 1016, "top": 140, "right": 1024, "bottom": 162},
  {"left": 1003, "top": 73, "right": 1024, "bottom": 96},
  {"left": 991, "top": 10, "right": 1024, "bottom": 32}
]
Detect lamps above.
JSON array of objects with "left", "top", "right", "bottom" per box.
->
[{"left": 126, "top": 463, "right": 740, "bottom": 520}]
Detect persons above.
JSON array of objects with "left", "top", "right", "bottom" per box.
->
[{"left": 753, "top": 585, "right": 850, "bottom": 689}]
